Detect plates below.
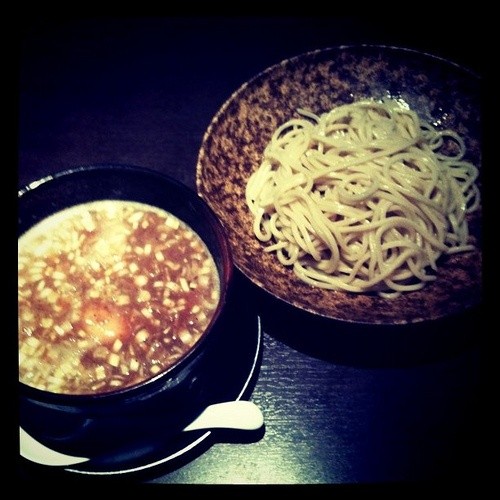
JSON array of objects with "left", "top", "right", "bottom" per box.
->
[{"left": 11, "top": 270, "right": 261, "bottom": 476}]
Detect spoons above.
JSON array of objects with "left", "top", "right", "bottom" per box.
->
[{"left": 19, "top": 400, "right": 264, "bottom": 467}]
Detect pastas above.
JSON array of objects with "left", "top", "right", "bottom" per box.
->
[{"left": 245, "top": 100, "right": 482, "bottom": 299}]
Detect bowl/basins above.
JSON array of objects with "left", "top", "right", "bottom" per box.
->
[
  {"left": 9, "top": 162, "right": 232, "bottom": 425},
  {"left": 196, "top": 43, "right": 500, "bottom": 329}
]
